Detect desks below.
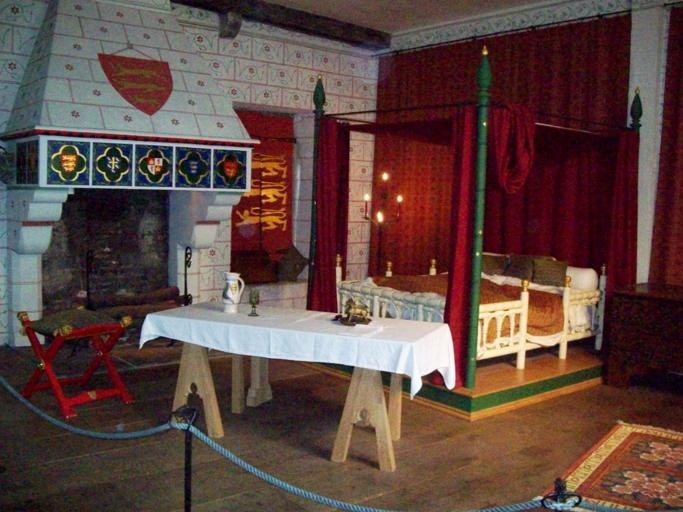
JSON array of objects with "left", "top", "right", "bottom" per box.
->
[{"left": 147, "top": 301, "right": 446, "bottom": 476}]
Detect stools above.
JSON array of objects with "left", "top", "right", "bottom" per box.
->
[{"left": 16, "top": 302, "right": 136, "bottom": 419}]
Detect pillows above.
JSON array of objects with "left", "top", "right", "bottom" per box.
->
[{"left": 480, "top": 255, "right": 569, "bottom": 289}]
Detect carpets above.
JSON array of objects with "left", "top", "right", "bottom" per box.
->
[{"left": 529, "top": 417, "right": 683, "bottom": 511}]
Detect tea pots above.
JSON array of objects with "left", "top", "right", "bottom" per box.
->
[{"left": 221, "top": 272, "right": 245, "bottom": 313}]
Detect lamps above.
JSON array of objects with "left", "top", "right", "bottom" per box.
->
[{"left": 362, "top": 170, "right": 403, "bottom": 275}]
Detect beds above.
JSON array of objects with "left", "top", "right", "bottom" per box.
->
[{"left": 335, "top": 250, "right": 612, "bottom": 389}]
